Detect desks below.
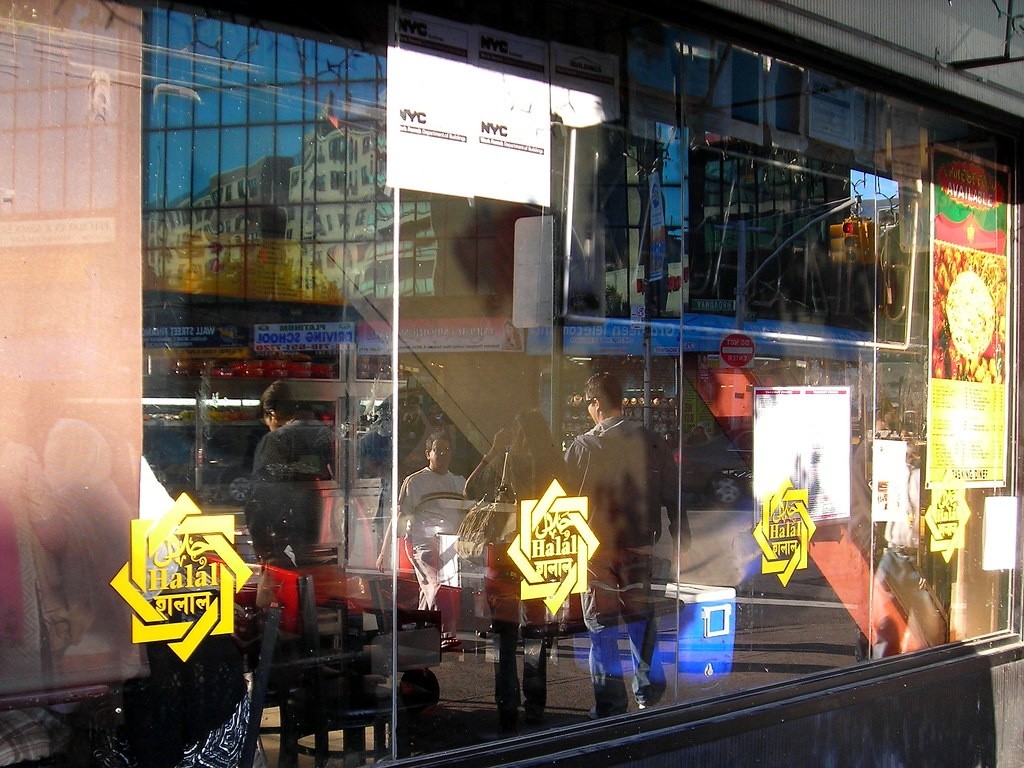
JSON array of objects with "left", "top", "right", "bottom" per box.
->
[
  {"left": 106, "top": 630, "right": 382, "bottom": 696},
  {"left": 314, "top": 573, "right": 685, "bottom": 638}
]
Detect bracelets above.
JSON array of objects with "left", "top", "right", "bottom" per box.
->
[{"left": 481, "top": 455, "right": 490, "bottom": 465}]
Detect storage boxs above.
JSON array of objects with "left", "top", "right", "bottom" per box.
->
[{"left": 572, "top": 582, "right": 736, "bottom": 685}]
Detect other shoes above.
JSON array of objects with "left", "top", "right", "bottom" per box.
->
[
  {"left": 441, "top": 638, "right": 463, "bottom": 648},
  {"left": 475, "top": 628, "right": 493, "bottom": 639}
]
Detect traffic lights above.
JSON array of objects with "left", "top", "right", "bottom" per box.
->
[
  {"left": 829, "top": 224, "right": 845, "bottom": 266},
  {"left": 842, "top": 220, "right": 862, "bottom": 266}
]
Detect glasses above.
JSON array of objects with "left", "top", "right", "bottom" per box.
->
[{"left": 585, "top": 399, "right": 597, "bottom": 408}]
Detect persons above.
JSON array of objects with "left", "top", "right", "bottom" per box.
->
[
  {"left": 560, "top": 370, "right": 694, "bottom": 718},
  {"left": 393, "top": 426, "right": 475, "bottom": 618},
  {"left": 244, "top": 379, "right": 337, "bottom": 557},
  {"left": 845, "top": 391, "right": 950, "bottom": 664},
  {"left": 461, "top": 409, "right": 573, "bottom": 715}
]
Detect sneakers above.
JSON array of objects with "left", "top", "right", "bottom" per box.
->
[
  {"left": 589, "top": 706, "right": 598, "bottom": 719},
  {"left": 639, "top": 704, "right": 647, "bottom": 710}
]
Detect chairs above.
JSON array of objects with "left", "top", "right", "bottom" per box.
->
[{"left": 0, "top": 564, "right": 475, "bottom": 768}]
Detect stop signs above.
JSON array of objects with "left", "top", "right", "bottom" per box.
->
[{"left": 720, "top": 331, "right": 755, "bottom": 369}]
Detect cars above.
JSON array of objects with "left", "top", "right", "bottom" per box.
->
[
  {"left": 672, "top": 427, "right": 753, "bottom": 506},
  {"left": 195, "top": 420, "right": 266, "bottom": 507}
]
